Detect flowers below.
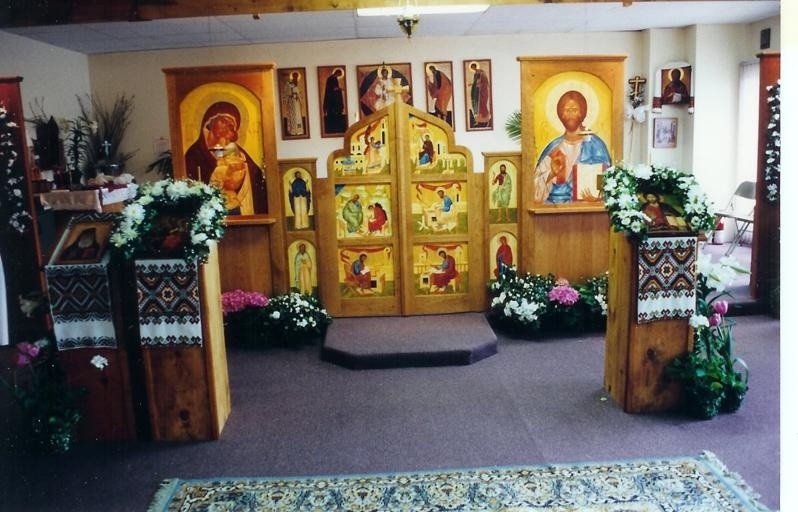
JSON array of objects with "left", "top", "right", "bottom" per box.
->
[
  {"left": 0, "top": 99, "right": 34, "bottom": 237},
  {"left": 108, "top": 176, "right": 229, "bottom": 266},
  {"left": 763, "top": 77, "right": 781, "bottom": 208},
  {"left": 220, "top": 283, "right": 333, "bottom": 349},
  {"left": 485, "top": 263, "right": 609, "bottom": 342},
  {"left": 603, "top": 158, "right": 720, "bottom": 241},
  {"left": 89, "top": 351, "right": 111, "bottom": 408},
  {"left": 663, "top": 250, "right": 754, "bottom": 421},
  {"left": 1, "top": 331, "right": 91, "bottom": 439}
]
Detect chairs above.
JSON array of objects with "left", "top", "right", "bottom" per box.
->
[{"left": 703, "top": 178, "right": 758, "bottom": 257}]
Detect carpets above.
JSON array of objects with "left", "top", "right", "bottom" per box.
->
[{"left": 145, "top": 448, "right": 778, "bottom": 512}]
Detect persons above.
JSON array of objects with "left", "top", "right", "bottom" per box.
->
[
  {"left": 60, "top": 228, "right": 99, "bottom": 260},
  {"left": 295, "top": 243, "right": 313, "bottom": 295},
  {"left": 492, "top": 163, "right": 512, "bottom": 222},
  {"left": 494, "top": 236, "right": 513, "bottom": 281},
  {"left": 288, "top": 172, "right": 311, "bottom": 230}
]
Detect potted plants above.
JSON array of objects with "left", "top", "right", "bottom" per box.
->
[{"left": 23, "top": 89, "right": 142, "bottom": 190}]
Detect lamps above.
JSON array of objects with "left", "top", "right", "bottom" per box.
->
[{"left": 396, "top": 0, "right": 420, "bottom": 40}]
